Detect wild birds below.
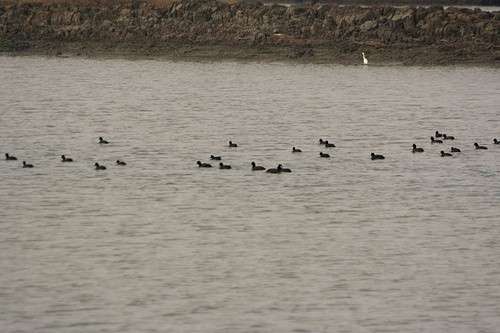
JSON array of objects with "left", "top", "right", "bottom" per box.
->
[
  {"left": 116, "top": 160, "right": 126, "bottom": 165},
  {"left": 99, "top": 137, "right": 108, "bottom": 143},
  {"left": 319, "top": 151, "right": 330, "bottom": 157},
  {"left": 4, "top": 152, "right": 17, "bottom": 160},
  {"left": 94, "top": 162, "right": 107, "bottom": 170},
  {"left": 22, "top": 161, "right": 33, "bottom": 167},
  {"left": 370, "top": 153, "right": 385, "bottom": 160},
  {"left": 291, "top": 147, "right": 301, "bottom": 153},
  {"left": 61, "top": 155, "right": 73, "bottom": 162},
  {"left": 318, "top": 138, "right": 335, "bottom": 147},
  {"left": 430, "top": 130, "right": 500, "bottom": 157},
  {"left": 228, "top": 140, "right": 237, "bottom": 147},
  {"left": 196, "top": 155, "right": 232, "bottom": 169},
  {"left": 411, "top": 143, "right": 424, "bottom": 153},
  {"left": 251, "top": 161, "right": 292, "bottom": 174}
]
